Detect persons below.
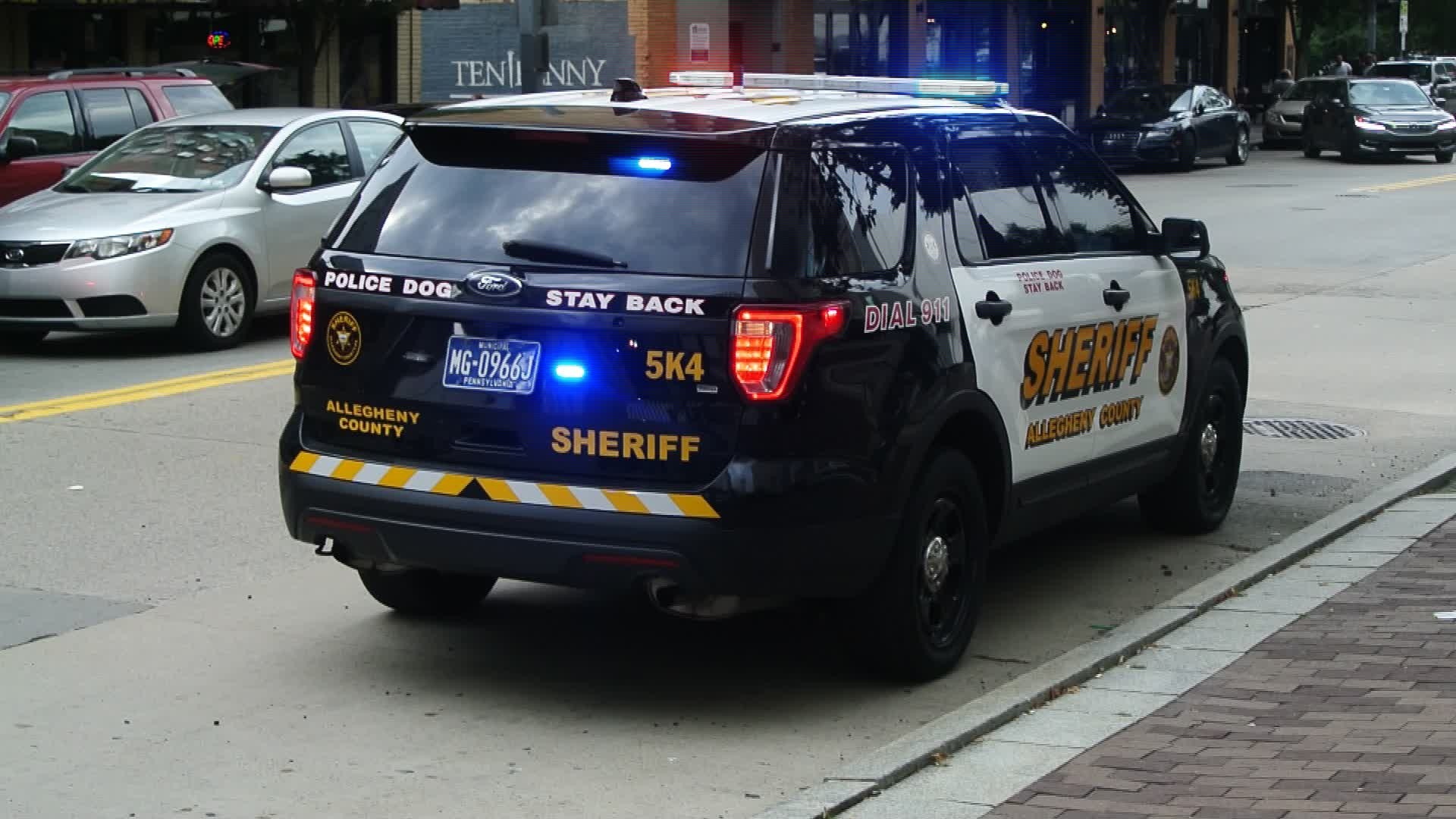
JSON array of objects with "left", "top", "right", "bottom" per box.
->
[
  {"left": 1274, "top": 68, "right": 1295, "bottom": 99},
  {"left": 1328, "top": 55, "right": 1353, "bottom": 76}
]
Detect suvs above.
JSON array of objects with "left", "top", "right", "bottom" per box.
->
[
  {"left": 0, "top": 63, "right": 239, "bottom": 213},
  {"left": 1360, "top": 54, "right": 1456, "bottom": 116},
  {"left": 276, "top": 67, "right": 1253, "bottom": 683}
]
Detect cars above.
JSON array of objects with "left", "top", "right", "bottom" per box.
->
[
  {"left": 1072, "top": 82, "right": 1252, "bottom": 173},
  {"left": 1300, "top": 77, "right": 1456, "bottom": 163},
  {"left": 0, "top": 106, "right": 409, "bottom": 354},
  {"left": 1261, "top": 75, "right": 1358, "bottom": 149}
]
